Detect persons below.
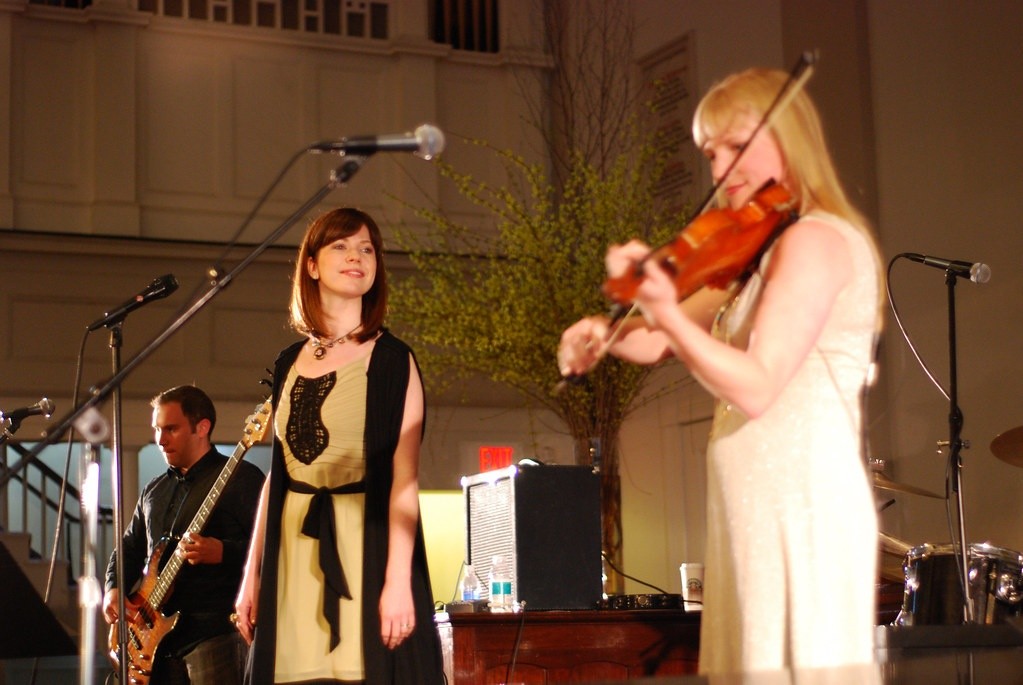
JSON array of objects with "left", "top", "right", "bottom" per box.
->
[
  {"left": 235, "top": 205, "right": 445, "bottom": 684},
  {"left": 558, "top": 71, "right": 885, "bottom": 684},
  {"left": 102, "top": 386, "right": 266, "bottom": 685}
]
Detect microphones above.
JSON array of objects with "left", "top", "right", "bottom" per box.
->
[
  {"left": 308, "top": 124, "right": 446, "bottom": 160},
  {"left": 0, "top": 398, "right": 55, "bottom": 421},
  {"left": 88, "top": 274, "right": 181, "bottom": 330},
  {"left": 905, "top": 253, "right": 991, "bottom": 283}
]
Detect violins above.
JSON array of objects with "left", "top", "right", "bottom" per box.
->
[{"left": 602, "top": 179, "right": 800, "bottom": 305}]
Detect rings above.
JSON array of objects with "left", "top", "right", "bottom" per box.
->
[
  {"left": 402, "top": 623, "right": 409, "bottom": 627},
  {"left": 230, "top": 613, "right": 238, "bottom": 623}
]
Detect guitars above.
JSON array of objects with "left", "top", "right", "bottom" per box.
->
[{"left": 107, "top": 365, "right": 273, "bottom": 685}]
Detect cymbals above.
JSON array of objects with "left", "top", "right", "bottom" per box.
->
[
  {"left": 990, "top": 424, "right": 1023, "bottom": 468},
  {"left": 870, "top": 472, "right": 945, "bottom": 500}
]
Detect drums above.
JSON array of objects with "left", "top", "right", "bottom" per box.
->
[{"left": 900, "top": 541, "right": 1023, "bottom": 624}]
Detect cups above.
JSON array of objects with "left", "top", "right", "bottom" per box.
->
[{"left": 680, "top": 562, "right": 704, "bottom": 611}]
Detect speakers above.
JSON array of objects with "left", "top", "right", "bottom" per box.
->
[{"left": 462, "top": 463, "right": 603, "bottom": 610}]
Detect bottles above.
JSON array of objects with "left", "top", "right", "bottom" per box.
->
[
  {"left": 488, "top": 555, "right": 513, "bottom": 613},
  {"left": 460, "top": 565, "right": 479, "bottom": 601}
]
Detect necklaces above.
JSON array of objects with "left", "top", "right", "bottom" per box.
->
[{"left": 312, "top": 324, "right": 362, "bottom": 360}]
